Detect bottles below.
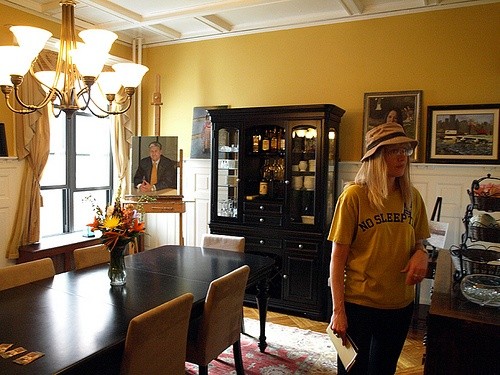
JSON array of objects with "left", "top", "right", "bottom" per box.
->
[
  {"left": 263, "top": 158, "right": 285, "bottom": 181},
  {"left": 252, "top": 127, "right": 286, "bottom": 152},
  {"left": 259, "top": 171, "right": 268, "bottom": 203}
]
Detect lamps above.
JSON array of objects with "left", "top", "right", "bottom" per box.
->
[{"left": 0, "top": 0, "right": 150, "bottom": 118}]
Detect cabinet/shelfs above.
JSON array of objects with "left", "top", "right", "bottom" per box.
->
[
  {"left": 205, "top": 103, "right": 346, "bottom": 326},
  {"left": 449, "top": 173, "right": 500, "bottom": 300}
]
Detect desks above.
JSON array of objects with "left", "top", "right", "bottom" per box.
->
[
  {"left": 16, "top": 228, "right": 144, "bottom": 272},
  {"left": 122, "top": 198, "right": 185, "bottom": 252},
  {"left": 421, "top": 248, "right": 500, "bottom": 375},
  {"left": 0, "top": 245, "right": 276, "bottom": 375}
]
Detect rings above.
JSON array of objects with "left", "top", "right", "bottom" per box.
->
[{"left": 413, "top": 274, "right": 417, "bottom": 277}]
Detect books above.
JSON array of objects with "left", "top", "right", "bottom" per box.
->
[{"left": 326, "top": 315, "right": 359, "bottom": 372}]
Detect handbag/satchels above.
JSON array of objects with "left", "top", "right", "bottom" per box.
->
[{"left": 425, "top": 197, "right": 449, "bottom": 248}]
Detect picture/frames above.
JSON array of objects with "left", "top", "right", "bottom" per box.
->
[
  {"left": 425, "top": 103, "right": 500, "bottom": 165},
  {"left": 362, "top": 89, "right": 423, "bottom": 163}
]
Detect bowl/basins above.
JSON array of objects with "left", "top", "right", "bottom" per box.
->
[
  {"left": 302, "top": 216, "right": 314, "bottom": 224},
  {"left": 460, "top": 274, "right": 500, "bottom": 307}
]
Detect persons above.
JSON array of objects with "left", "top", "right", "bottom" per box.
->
[
  {"left": 133, "top": 141, "right": 177, "bottom": 190},
  {"left": 327, "top": 122, "right": 432, "bottom": 375}
]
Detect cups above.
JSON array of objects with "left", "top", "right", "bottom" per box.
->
[
  {"left": 292, "top": 165, "right": 299, "bottom": 171},
  {"left": 304, "top": 176, "right": 315, "bottom": 191},
  {"left": 217, "top": 159, "right": 238, "bottom": 169},
  {"left": 227, "top": 176, "right": 236, "bottom": 185},
  {"left": 309, "top": 160, "right": 316, "bottom": 172},
  {"left": 219, "top": 198, "right": 237, "bottom": 215},
  {"left": 292, "top": 176, "right": 303, "bottom": 190},
  {"left": 299, "top": 160, "right": 307, "bottom": 171}
]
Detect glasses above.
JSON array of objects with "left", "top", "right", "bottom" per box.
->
[{"left": 384, "top": 148, "right": 413, "bottom": 156}]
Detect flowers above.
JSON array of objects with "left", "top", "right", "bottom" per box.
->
[{"left": 82, "top": 169, "right": 159, "bottom": 282}]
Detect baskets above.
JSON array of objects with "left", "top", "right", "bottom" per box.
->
[
  {"left": 467, "top": 175, "right": 500, "bottom": 210},
  {"left": 468, "top": 218, "right": 500, "bottom": 242},
  {"left": 448, "top": 244, "right": 500, "bottom": 275}
]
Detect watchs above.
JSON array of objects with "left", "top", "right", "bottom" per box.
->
[{"left": 416, "top": 247, "right": 430, "bottom": 255}]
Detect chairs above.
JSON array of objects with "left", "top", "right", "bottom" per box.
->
[{"left": 0, "top": 234, "right": 250, "bottom": 375}]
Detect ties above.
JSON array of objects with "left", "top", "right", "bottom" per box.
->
[{"left": 151, "top": 161, "right": 157, "bottom": 185}]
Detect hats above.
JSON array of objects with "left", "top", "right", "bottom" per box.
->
[{"left": 361, "top": 122, "right": 417, "bottom": 163}]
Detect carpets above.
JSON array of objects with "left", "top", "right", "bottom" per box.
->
[{"left": 185, "top": 317, "right": 337, "bottom": 375}]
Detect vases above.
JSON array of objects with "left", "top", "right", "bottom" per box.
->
[{"left": 108, "top": 246, "right": 127, "bottom": 285}]
